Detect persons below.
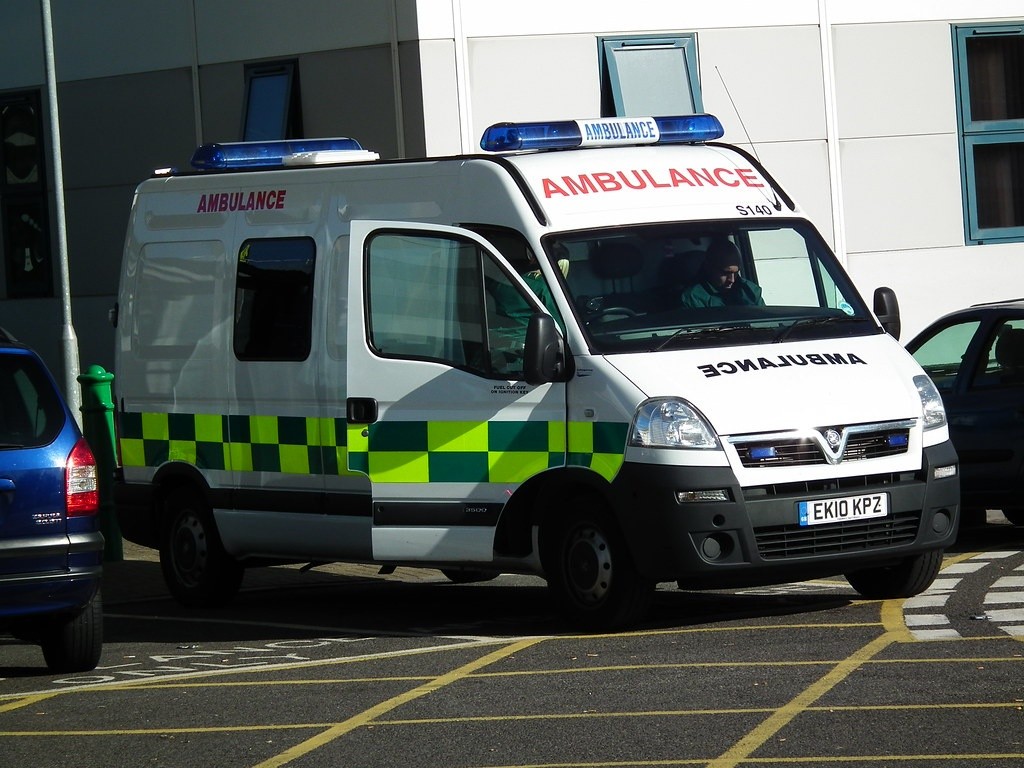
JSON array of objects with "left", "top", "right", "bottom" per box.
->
[
  {"left": 681, "top": 238, "right": 766, "bottom": 320},
  {"left": 480, "top": 239, "right": 564, "bottom": 372}
]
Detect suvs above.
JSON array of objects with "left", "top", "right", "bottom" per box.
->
[{"left": 3, "top": 327, "right": 113, "bottom": 668}]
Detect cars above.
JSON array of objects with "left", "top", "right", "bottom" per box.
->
[{"left": 903, "top": 295, "right": 1024, "bottom": 548}]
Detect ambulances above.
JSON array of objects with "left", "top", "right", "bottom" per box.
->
[{"left": 123, "top": 113, "right": 963, "bottom": 625}]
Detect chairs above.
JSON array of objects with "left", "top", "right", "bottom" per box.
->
[
  {"left": 662, "top": 251, "right": 709, "bottom": 296},
  {"left": 575, "top": 236, "right": 676, "bottom": 316},
  {"left": 973, "top": 328, "right": 1023, "bottom": 403}
]
